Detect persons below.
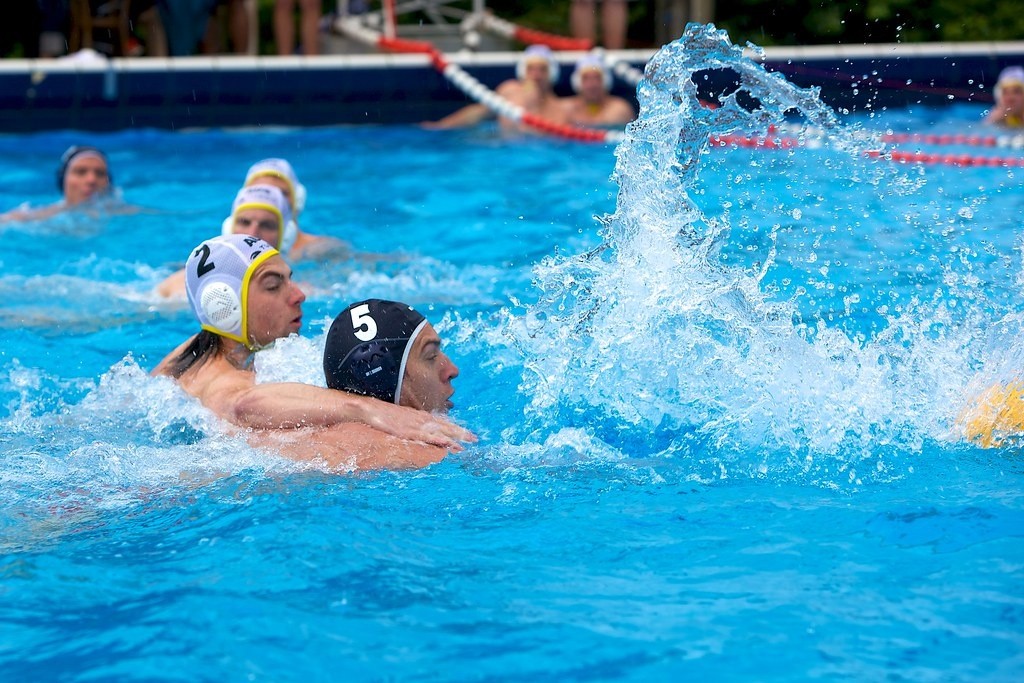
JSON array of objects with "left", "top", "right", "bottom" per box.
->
[
  {"left": 423, "top": 43, "right": 634, "bottom": 138},
  {"left": 984, "top": 67, "right": 1024, "bottom": 128},
  {"left": 0, "top": 143, "right": 114, "bottom": 222},
  {"left": 154, "top": 158, "right": 345, "bottom": 298},
  {"left": 149, "top": 233, "right": 477, "bottom": 469}
]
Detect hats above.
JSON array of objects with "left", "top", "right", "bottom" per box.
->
[
  {"left": 56, "top": 144, "right": 114, "bottom": 194},
  {"left": 516, "top": 45, "right": 559, "bottom": 81},
  {"left": 243, "top": 158, "right": 306, "bottom": 216},
  {"left": 221, "top": 185, "right": 297, "bottom": 252},
  {"left": 994, "top": 67, "right": 1024, "bottom": 101},
  {"left": 323, "top": 300, "right": 427, "bottom": 404},
  {"left": 185, "top": 234, "right": 279, "bottom": 344},
  {"left": 571, "top": 58, "right": 612, "bottom": 91}
]
